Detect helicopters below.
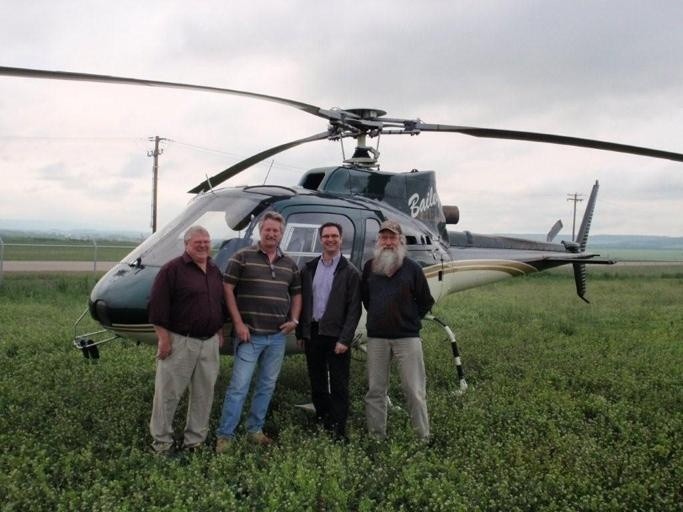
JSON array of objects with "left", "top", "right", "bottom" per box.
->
[{"left": 0, "top": 66, "right": 682, "bottom": 412}]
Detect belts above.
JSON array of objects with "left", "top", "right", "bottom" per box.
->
[
  {"left": 167, "top": 326, "right": 214, "bottom": 340},
  {"left": 250, "top": 328, "right": 281, "bottom": 337}
]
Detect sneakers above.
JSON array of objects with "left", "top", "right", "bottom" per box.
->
[
  {"left": 215, "top": 435, "right": 235, "bottom": 453},
  {"left": 248, "top": 432, "right": 278, "bottom": 449}
]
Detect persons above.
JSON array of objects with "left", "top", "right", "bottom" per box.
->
[
  {"left": 292, "top": 222, "right": 364, "bottom": 445},
  {"left": 146, "top": 225, "right": 228, "bottom": 460},
  {"left": 360, "top": 221, "right": 436, "bottom": 449},
  {"left": 214, "top": 211, "right": 303, "bottom": 454}
]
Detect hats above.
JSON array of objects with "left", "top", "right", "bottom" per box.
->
[{"left": 378, "top": 220, "right": 402, "bottom": 235}]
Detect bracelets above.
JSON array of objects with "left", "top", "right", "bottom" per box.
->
[{"left": 289, "top": 318, "right": 300, "bottom": 327}]
